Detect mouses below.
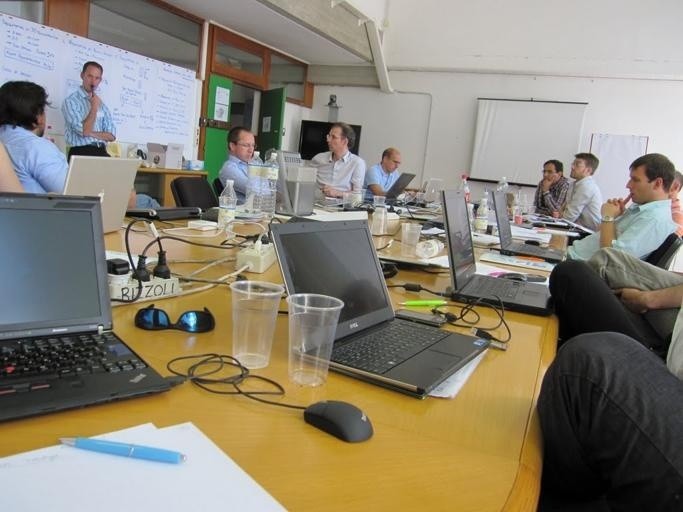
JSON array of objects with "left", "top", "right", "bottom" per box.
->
[
  {"left": 525, "top": 239, "right": 540, "bottom": 247},
  {"left": 532, "top": 222, "right": 546, "bottom": 227},
  {"left": 497, "top": 273, "right": 526, "bottom": 281},
  {"left": 301, "top": 399, "right": 375, "bottom": 441}
]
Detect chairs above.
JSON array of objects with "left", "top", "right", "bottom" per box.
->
[
  {"left": 170, "top": 176, "right": 217, "bottom": 209},
  {"left": 643, "top": 232, "right": 682, "bottom": 270},
  {"left": 211, "top": 177, "right": 224, "bottom": 204}
]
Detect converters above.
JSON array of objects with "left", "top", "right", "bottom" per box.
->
[{"left": 106, "top": 253, "right": 131, "bottom": 276}]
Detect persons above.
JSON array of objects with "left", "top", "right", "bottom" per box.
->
[
  {"left": 62, "top": 62, "right": 117, "bottom": 165},
  {"left": 559, "top": 153, "right": 604, "bottom": 233},
  {"left": 534, "top": 160, "right": 570, "bottom": 218},
  {"left": 218, "top": 127, "right": 271, "bottom": 206},
  {"left": 536, "top": 331, "right": 683, "bottom": 512},
  {"left": 669, "top": 171, "right": 683, "bottom": 237},
  {"left": 363, "top": 147, "right": 401, "bottom": 201},
  {"left": 311, "top": 122, "right": 366, "bottom": 201},
  {"left": 0, "top": 81, "right": 161, "bottom": 210},
  {"left": 567, "top": 154, "right": 675, "bottom": 262},
  {"left": 590, "top": 247, "right": 683, "bottom": 385}
]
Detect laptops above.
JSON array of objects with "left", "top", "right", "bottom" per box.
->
[
  {"left": 61, "top": 155, "right": 141, "bottom": 234},
  {"left": 0, "top": 195, "right": 172, "bottom": 419},
  {"left": 439, "top": 189, "right": 551, "bottom": 317},
  {"left": 365, "top": 172, "right": 416, "bottom": 201},
  {"left": 492, "top": 188, "right": 565, "bottom": 263},
  {"left": 423, "top": 178, "right": 443, "bottom": 203},
  {"left": 270, "top": 218, "right": 494, "bottom": 394}
]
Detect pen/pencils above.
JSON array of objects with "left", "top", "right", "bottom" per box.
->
[
  {"left": 514, "top": 255, "right": 545, "bottom": 262},
  {"left": 59, "top": 438, "right": 187, "bottom": 464},
  {"left": 489, "top": 272, "right": 507, "bottom": 276},
  {"left": 399, "top": 300, "right": 448, "bottom": 306}
]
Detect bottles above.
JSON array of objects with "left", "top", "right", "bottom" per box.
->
[
  {"left": 246, "top": 150, "right": 279, "bottom": 219},
  {"left": 218, "top": 178, "right": 239, "bottom": 227},
  {"left": 417, "top": 239, "right": 446, "bottom": 257},
  {"left": 461, "top": 174, "right": 531, "bottom": 239}
]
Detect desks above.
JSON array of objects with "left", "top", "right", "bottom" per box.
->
[
  {"left": 137, "top": 165, "right": 208, "bottom": 208},
  {"left": 1, "top": 204, "right": 570, "bottom": 512}
]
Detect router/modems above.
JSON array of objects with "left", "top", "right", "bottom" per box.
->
[{"left": 187, "top": 219, "right": 218, "bottom": 231}]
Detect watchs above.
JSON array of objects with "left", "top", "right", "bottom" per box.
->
[{"left": 601, "top": 216, "right": 613, "bottom": 222}]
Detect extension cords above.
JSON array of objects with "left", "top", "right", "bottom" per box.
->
[
  {"left": 109, "top": 274, "right": 182, "bottom": 303},
  {"left": 236, "top": 242, "right": 276, "bottom": 274},
  {"left": 107, "top": 271, "right": 133, "bottom": 284}
]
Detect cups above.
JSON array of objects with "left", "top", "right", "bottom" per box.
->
[
  {"left": 401, "top": 222, "right": 423, "bottom": 245},
  {"left": 227, "top": 279, "right": 285, "bottom": 369},
  {"left": 287, "top": 292, "right": 345, "bottom": 385},
  {"left": 342, "top": 188, "right": 388, "bottom": 235},
  {"left": 417, "top": 192, "right": 425, "bottom": 201}
]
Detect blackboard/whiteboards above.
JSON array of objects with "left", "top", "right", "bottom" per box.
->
[{"left": 0, "top": 12, "right": 203, "bottom": 161}]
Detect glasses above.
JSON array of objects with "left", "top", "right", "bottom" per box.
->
[
  {"left": 326, "top": 134, "right": 345, "bottom": 140},
  {"left": 234, "top": 142, "right": 258, "bottom": 150},
  {"left": 134, "top": 302, "right": 217, "bottom": 334}
]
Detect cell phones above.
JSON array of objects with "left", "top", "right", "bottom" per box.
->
[{"left": 393, "top": 308, "right": 448, "bottom": 327}]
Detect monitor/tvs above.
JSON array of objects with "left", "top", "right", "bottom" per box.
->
[{"left": 299, "top": 120, "right": 362, "bottom": 163}]
